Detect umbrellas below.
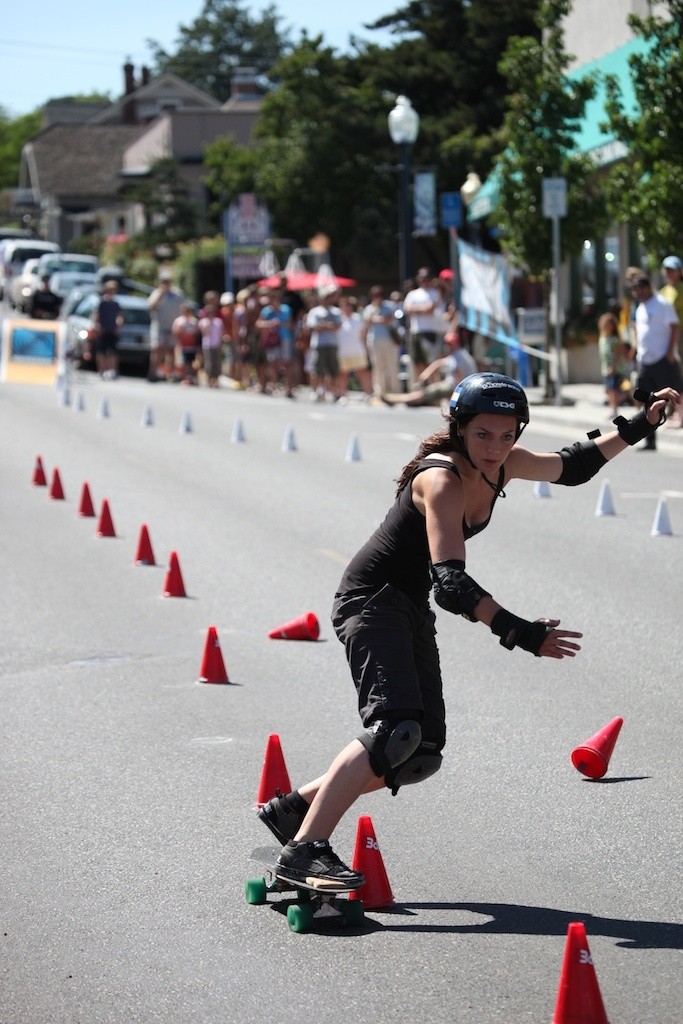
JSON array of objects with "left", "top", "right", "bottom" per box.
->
[{"left": 258, "top": 272, "right": 356, "bottom": 292}]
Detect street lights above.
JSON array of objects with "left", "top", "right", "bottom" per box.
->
[{"left": 387, "top": 95, "right": 423, "bottom": 307}]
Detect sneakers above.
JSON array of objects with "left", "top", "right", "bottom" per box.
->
[
  {"left": 257, "top": 789, "right": 300, "bottom": 847},
  {"left": 274, "top": 839, "right": 367, "bottom": 890}
]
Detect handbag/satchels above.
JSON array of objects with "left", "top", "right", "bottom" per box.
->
[{"left": 258, "top": 326, "right": 280, "bottom": 348}]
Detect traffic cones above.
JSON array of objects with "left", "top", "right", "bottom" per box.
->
[
  {"left": 571, "top": 717, "right": 624, "bottom": 780},
  {"left": 268, "top": 613, "right": 321, "bottom": 642},
  {"left": 594, "top": 483, "right": 616, "bottom": 517},
  {"left": 96, "top": 498, "right": 116, "bottom": 537},
  {"left": 347, "top": 814, "right": 397, "bottom": 912},
  {"left": 134, "top": 524, "right": 158, "bottom": 567},
  {"left": 61, "top": 385, "right": 363, "bottom": 465},
  {"left": 550, "top": 922, "right": 614, "bottom": 1024},
  {"left": 162, "top": 551, "right": 188, "bottom": 598},
  {"left": 538, "top": 479, "right": 552, "bottom": 500},
  {"left": 48, "top": 468, "right": 65, "bottom": 501},
  {"left": 198, "top": 626, "right": 230, "bottom": 684},
  {"left": 78, "top": 482, "right": 95, "bottom": 517},
  {"left": 31, "top": 456, "right": 48, "bottom": 487},
  {"left": 650, "top": 499, "right": 676, "bottom": 537},
  {"left": 252, "top": 734, "right": 293, "bottom": 808}
]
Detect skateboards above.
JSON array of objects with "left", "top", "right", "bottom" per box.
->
[{"left": 244, "top": 846, "right": 366, "bottom": 933}]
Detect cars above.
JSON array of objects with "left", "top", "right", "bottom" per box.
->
[{"left": 2, "top": 238, "right": 161, "bottom": 377}]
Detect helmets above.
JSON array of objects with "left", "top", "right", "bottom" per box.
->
[{"left": 450, "top": 372, "right": 529, "bottom": 425}]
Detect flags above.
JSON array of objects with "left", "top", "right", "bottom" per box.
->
[{"left": 447, "top": 229, "right": 521, "bottom": 347}]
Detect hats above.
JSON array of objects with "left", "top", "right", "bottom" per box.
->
[
  {"left": 444, "top": 334, "right": 460, "bottom": 344},
  {"left": 439, "top": 270, "right": 454, "bottom": 281},
  {"left": 417, "top": 268, "right": 429, "bottom": 277},
  {"left": 662, "top": 256, "right": 681, "bottom": 270}
]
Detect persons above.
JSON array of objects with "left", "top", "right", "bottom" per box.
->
[
  {"left": 146, "top": 271, "right": 183, "bottom": 381},
  {"left": 173, "top": 270, "right": 479, "bottom": 406},
  {"left": 600, "top": 256, "right": 683, "bottom": 417},
  {"left": 260, "top": 370, "right": 679, "bottom": 891},
  {"left": 92, "top": 281, "right": 125, "bottom": 376}
]
